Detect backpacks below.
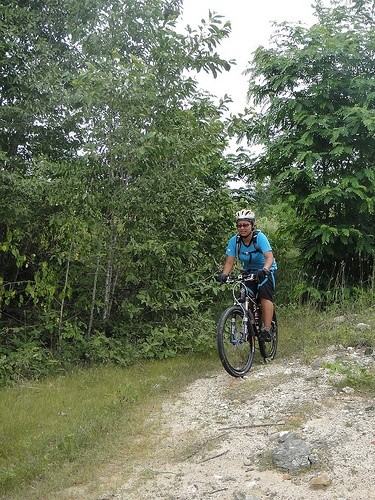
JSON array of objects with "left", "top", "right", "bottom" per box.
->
[{"left": 236, "top": 229, "right": 278, "bottom": 272}]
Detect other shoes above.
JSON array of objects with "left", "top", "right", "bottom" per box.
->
[{"left": 260, "top": 325, "right": 272, "bottom": 342}]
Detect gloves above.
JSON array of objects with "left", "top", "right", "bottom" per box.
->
[
  {"left": 216, "top": 275, "right": 226, "bottom": 283},
  {"left": 254, "top": 269, "right": 267, "bottom": 283}
]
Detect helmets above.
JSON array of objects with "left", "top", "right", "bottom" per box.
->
[{"left": 235, "top": 209, "right": 256, "bottom": 224}]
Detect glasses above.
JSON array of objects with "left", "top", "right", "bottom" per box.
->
[{"left": 236, "top": 222, "right": 252, "bottom": 228}]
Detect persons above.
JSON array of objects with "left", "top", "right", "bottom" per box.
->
[{"left": 216, "top": 209, "right": 277, "bottom": 341}]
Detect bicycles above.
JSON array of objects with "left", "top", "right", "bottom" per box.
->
[{"left": 212, "top": 273, "right": 280, "bottom": 379}]
